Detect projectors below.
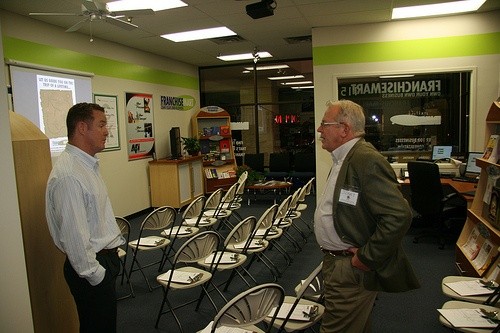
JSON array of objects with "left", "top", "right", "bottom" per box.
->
[{"left": 246, "top": 2, "right": 274, "bottom": 20}]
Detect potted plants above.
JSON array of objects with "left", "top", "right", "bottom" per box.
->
[{"left": 181, "top": 136, "right": 201, "bottom": 156}]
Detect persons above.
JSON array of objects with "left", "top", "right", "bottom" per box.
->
[
  {"left": 314, "top": 99, "right": 422, "bottom": 333},
  {"left": 45, "top": 102, "right": 126, "bottom": 333}
]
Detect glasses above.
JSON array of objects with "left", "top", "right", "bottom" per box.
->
[{"left": 319, "top": 121, "right": 345, "bottom": 127}]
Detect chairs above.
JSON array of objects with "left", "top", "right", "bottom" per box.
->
[
  {"left": 113, "top": 171, "right": 324, "bottom": 333},
  {"left": 438, "top": 248, "right": 500, "bottom": 333},
  {"left": 406, "top": 160, "right": 466, "bottom": 249},
  {"left": 237, "top": 152, "right": 316, "bottom": 194}
]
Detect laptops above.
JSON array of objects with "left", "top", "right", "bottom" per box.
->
[{"left": 431, "top": 146, "right": 452, "bottom": 163}]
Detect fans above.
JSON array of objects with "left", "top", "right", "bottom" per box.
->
[{"left": 28, "top": 0, "right": 138, "bottom": 42}]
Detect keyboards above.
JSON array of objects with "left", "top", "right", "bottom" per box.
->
[{"left": 452, "top": 177, "right": 479, "bottom": 183}]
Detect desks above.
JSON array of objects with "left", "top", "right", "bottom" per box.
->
[
  {"left": 245, "top": 180, "right": 293, "bottom": 206},
  {"left": 399, "top": 175, "right": 478, "bottom": 210}
]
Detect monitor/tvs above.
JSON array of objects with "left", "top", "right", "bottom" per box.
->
[
  {"left": 466, "top": 152, "right": 485, "bottom": 174},
  {"left": 170, "top": 127, "right": 182, "bottom": 160}
]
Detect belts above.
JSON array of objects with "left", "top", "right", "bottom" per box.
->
[
  {"left": 318, "top": 247, "right": 353, "bottom": 257},
  {"left": 99, "top": 248, "right": 118, "bottom": 254}
]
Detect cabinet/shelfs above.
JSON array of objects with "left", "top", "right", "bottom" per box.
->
[
  {"left": 148, "top": 153, "right": 205, "bottom": 215},
  {"left": 456, "top": 102, "right": 499, "bottom": 284},
  {"left": 192, "top": 106, "right": 239, "bottom": 194}
]
